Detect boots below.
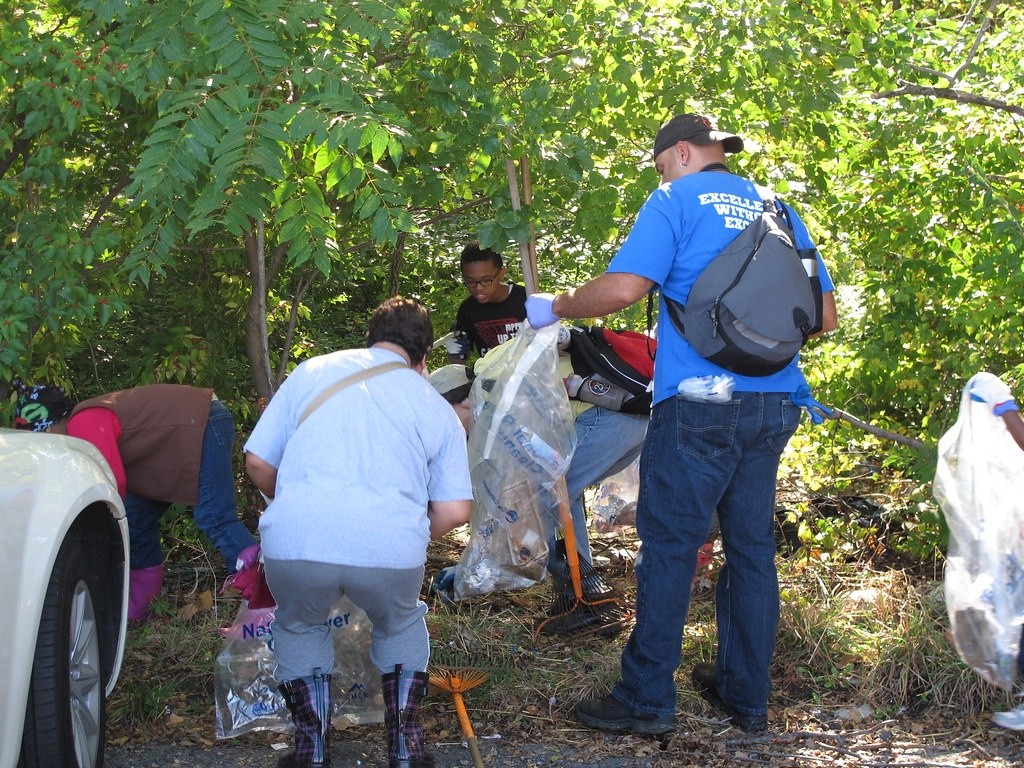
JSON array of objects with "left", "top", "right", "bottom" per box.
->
[
  {"left": 126, "top": 564, "right": 164, "bottom": 618},
  {"left": 381, "top": 663, "right": 436, "bottom": 768},
  {"left": 276, "top": 667, "right": 332, "bottom": 767},
  {"left": 218, "top": 544, "right": 275, "bottom": 638}
]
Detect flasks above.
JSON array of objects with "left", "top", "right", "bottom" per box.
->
[{"left": 796, "top": 246, "right": 819, "bottom": 293}]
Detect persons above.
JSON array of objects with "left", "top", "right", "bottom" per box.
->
[
  {"left": 242, "top": 291, "right": 474, "bottom": 766},
  {"left": 12, "top": 372, "right": 264, "bottom": 627},
  {"left": 446, "top": 245, "right": 534, "bottom": 370},
  {"left": 524, "top": 111, "right": 844, "bottom": 740},
  {"left": 425, "top": 323, "right": 661, "bottom": 636},
  {"left": 961, "top": 367, "right": 1023, "bottom": 736}
]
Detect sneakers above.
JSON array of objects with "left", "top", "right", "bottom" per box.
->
[
  {"left": 533, "top": 599, "right": 622, "bottom": 639},
  {"left": 691, "top": 662, "right": 768, "bottom": 733},
  {"left": 574, "top": 694, "right": 677, "bottom": 736},
  {"left": 989, "top": 702, "right": 1024, "bottom": 731}
]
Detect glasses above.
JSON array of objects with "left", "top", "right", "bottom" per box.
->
[{"left": 463, "top": 265, "right": 503, "bottom": 289}]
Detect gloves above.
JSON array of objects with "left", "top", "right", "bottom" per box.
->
[
  {"left": 524, "top": 292, "right": 560, "bottom": 331},
  {"left": 430, "top": 565, "right": 459, "bottom": 602},
  {"left": 970, "top": 369, "right": 1019, "bottom": 415}
]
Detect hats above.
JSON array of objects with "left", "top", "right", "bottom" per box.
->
[
  {"left": 12, "top": 385, "right": 71, "bottom": 432},
  {"left": 653, "top": 113, "right": 744, "bottom": 162}
]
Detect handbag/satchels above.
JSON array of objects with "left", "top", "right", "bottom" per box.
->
[{"left": 232, "top": 554, "right": 275, "bottom": 610}]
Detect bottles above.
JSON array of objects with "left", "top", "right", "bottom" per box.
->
[{"left": 560, "top": 372, "right": 625, "bottom": 412}]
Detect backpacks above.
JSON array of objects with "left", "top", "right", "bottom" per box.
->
[
  {"left": 662, "top": 181, "right": 824, "bottom": 378},
  {"left": 482, "top": 325, "right": 658, "bottom": 415}
]
